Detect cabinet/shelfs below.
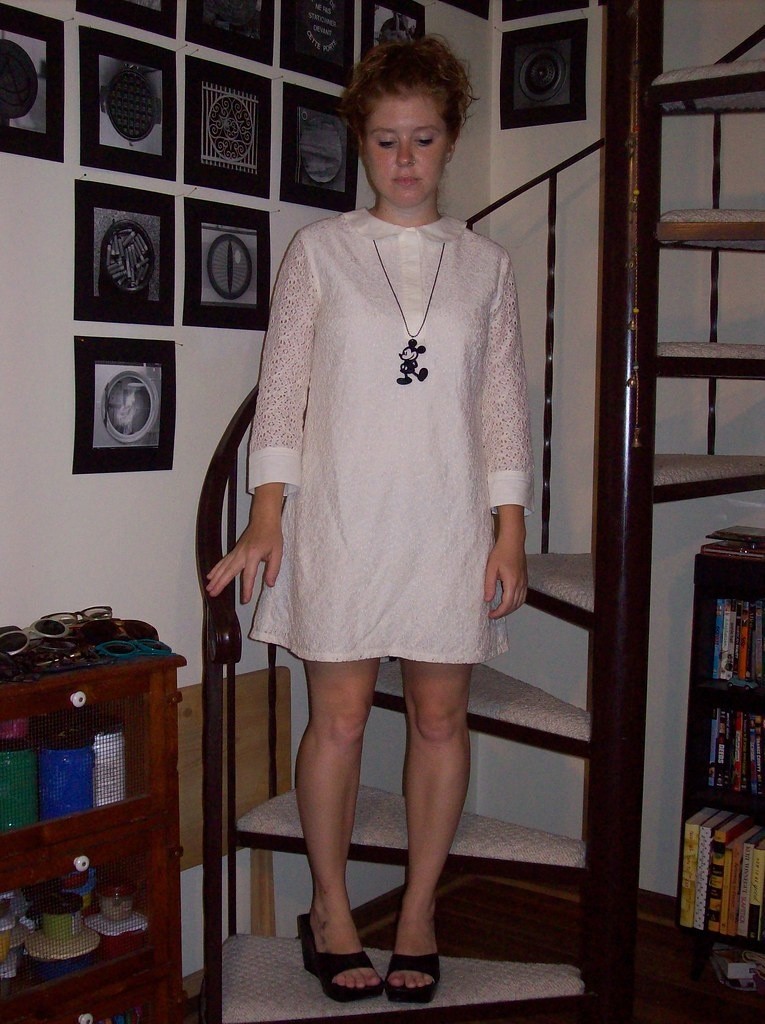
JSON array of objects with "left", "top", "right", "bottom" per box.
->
[
  {"left": 673, "top": 554, "right": 765, "bottom": 981},
  {"left": 1, "top": 654, "right": 187, "bottom": 1024}
]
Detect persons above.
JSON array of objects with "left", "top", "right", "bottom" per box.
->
[{"left": 206, "top": 37, "right": 532, "bottom": 1002}]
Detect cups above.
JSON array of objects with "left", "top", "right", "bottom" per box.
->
[
  {"left": 0, "top": 898, "right": 29, "bottom": 963},
  {"left": 94, "top": 879, "right": 135, "bottom": 919}
]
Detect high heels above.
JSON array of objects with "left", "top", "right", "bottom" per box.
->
[
  {"left": 296, "top": 911, "right": 384, "bottom": 1003},
  {"left": 385, "top": 951, "right": 440, "bottom": 1003}
]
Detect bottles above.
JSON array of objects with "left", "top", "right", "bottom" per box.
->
[{"left": 39, "top": 891, "right": 84, "bottom": 940}]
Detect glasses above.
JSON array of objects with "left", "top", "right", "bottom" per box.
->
[{"left": 0, "top": 605, "right": 175, "bottom": 684}]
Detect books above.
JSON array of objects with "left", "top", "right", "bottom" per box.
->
[{"left": 680, "top": 598, "right": 765, "bottom": 941}]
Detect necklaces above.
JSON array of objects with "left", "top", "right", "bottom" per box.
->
[{"left": 374, "top": 239, "right": 444, "bottom": 384}]
